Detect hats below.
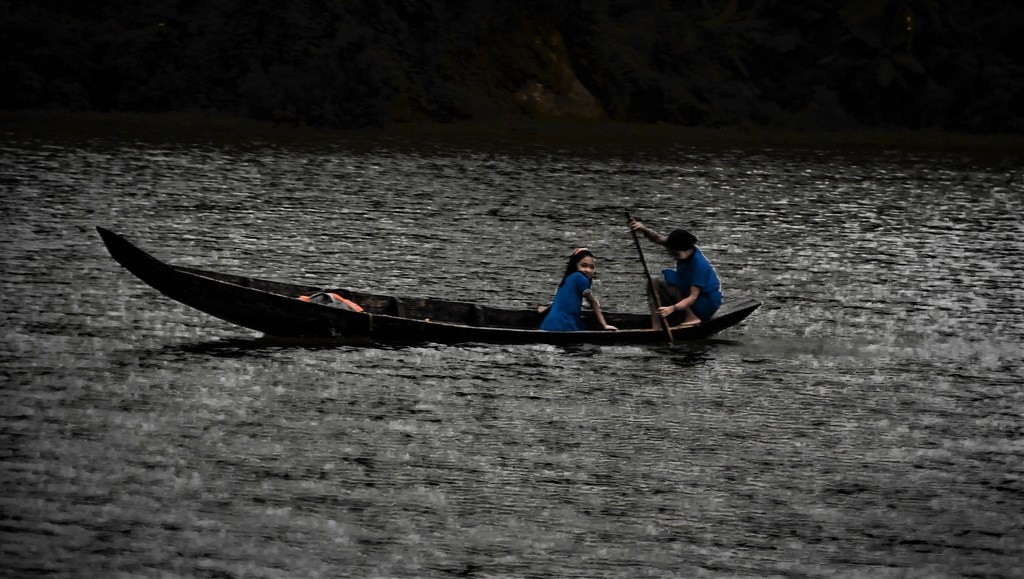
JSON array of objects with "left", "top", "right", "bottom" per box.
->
[{"left": 666, "top": 228, "right": 698, "bottom": 251}]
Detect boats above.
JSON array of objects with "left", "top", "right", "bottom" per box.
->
[{"left": 95, "top": 224, "right": 762, "bottom": 343}]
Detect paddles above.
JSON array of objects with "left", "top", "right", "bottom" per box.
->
[{"left": 622, "top": 209, "right": 681, "bottom": 347}]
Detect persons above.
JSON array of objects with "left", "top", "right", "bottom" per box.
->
[
  {"left": 540, "top": 248, "right": 619, "bottom": 331},
  {"left": 628, "top": 217, "right": 722, "bottom": 329}
]
